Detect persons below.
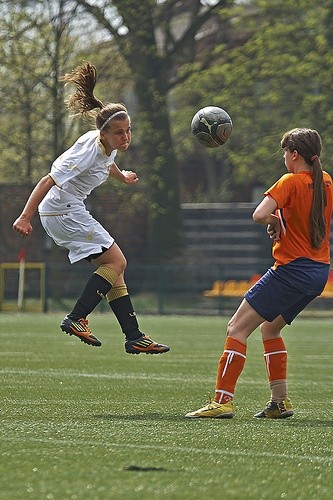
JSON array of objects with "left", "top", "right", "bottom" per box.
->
[
  {"left": 185, "top": 128, "right": 333, "bottom": 418},
  {"left": 13, "top": 60, "right": 170, "bottom": 354}
]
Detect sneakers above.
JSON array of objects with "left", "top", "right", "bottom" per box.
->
[
  {"left": 185, "top": 400, "right": 236, "bottom": 419},
  {"left": 252, "top": 397, "right": 293, "bottom": 419},
  {"left": 60, "top": 316, "right": 102, "bottom": 348},
  {"left": 124, "top": 335, "right": 169, "bottom": 355}
]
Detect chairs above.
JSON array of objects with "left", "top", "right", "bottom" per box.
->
[{"left": 202, "top": 269, "right": 333, "bottom": 315}]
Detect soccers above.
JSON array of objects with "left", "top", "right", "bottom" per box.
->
[{"left": 189, "top": 105, "right": 234, "bottom": 149}]
